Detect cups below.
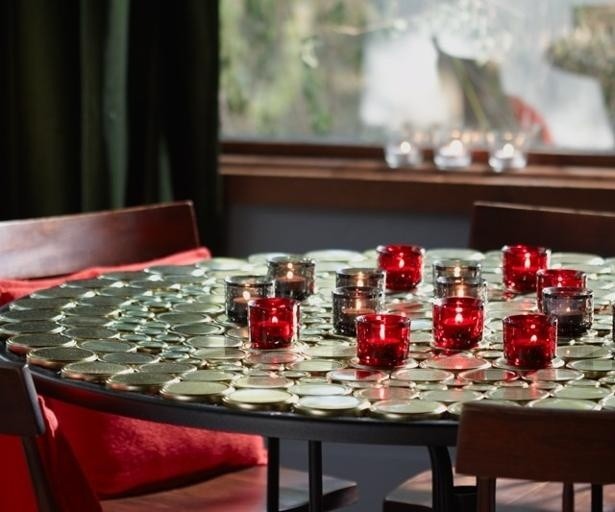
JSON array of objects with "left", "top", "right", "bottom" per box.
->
[
  {"left": 223, "top": 273, "right": 275, "bottom": 323},
  {"left": 386, "top": 124, "right": 538, "bottom": 173},
  {"left": 431, "top": 258, "right": 489, "bottom": 316},
  {"left": 502, "top": 243, "right": 595, "bottom": 336},
  {"left": 324, "top": 243, "right": 424, "bottom": 331},
  {"left": 265, "top": 255, "right": 315, "bottom": 297}
]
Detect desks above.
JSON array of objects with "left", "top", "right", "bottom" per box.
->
[{"left": 1, "top": 248, "right": 615, "bottom": 511}]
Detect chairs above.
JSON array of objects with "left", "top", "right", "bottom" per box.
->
[
  {"left": 381, "top": 202, "right": 614, "bottom": 512},
  {"left": 1, "top": 199, "right": 358, "bottom": 512},
  {"left": 453, "top": 399, "right": 615, "bottom": 511}
]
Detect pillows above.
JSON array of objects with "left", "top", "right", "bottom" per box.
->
[{"left": 0, "top": 245, "right": 270, "bottom": 500}]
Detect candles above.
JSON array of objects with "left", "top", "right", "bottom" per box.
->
[
  {"left": 437, "top": 275, "right": 488, "bottom": 300},
  {"left": 225, "top": 275, "right": 272, "bottom": 324},
  {"left": 337, "top": 268, "right": 384, "bottom": 288},
  {"left": 331, "top": 288, "right": 381, "bottom": 334},
  {"left": 271, "top": 257, "right": 315, "bottom": 300},
  {"left": 536, "top": 268, "right": 587, "bottom": 311},
  {"left": 380, "top": 244, "right": 422, "bottom": 291},
  {"left": 501, "top": 246, "right": 550, "bottom": 294},
  {"left": 541, "top": 287, "right": 593, "bottom": 341},
  {"left": 432, "top": 258, "right": 483, "bottom": 286}
]
